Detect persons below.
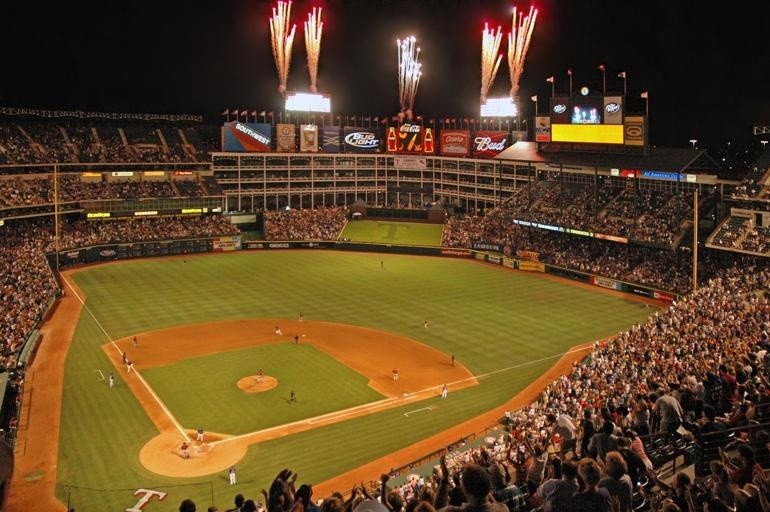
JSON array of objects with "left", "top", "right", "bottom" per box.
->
[
  {"left": 127, "top": 359, "right": 135, "bottom": 373},
  {"left": 299, "top": 311, "right": 304, "bottom": 322},
  {"left": 392, "top": 368, "right": 400, "bottom": 383},
  {"left": 424, "top": 318, "right": 428, "bottom": 329},
  {"left": 294, "top": 333, "right": 299, "bottom": 345},
  {"left": 290, "top": 389, "right": 296, "bottom": 401},
  {"left": 256, "top": 367, "right": 264, "bottom": 383},
  {"left": 2, "top": 208, "right": 60, "bottom": 290},
  {"left": 122, "top": 351, "right": 126, "bottom": 364},
  {"left": 196, "top": 425, "right": 205, "bottom": 442},
  {"left": 540, "top": 241, "right": 721, "bottom": 290},
  {"left": 109, "top": 374, "right": 114, "bottom": 387},
  {"left": 2, "top": 174, "right": 224, "bottom": 208},
  {"left": 0, "top": 247, "right": 61, "bottom": 372},
  {"left": 441, "top": 292, "right": 769, "bottom": 510},
  {"left": 441, "top": 211, "right": 500, "bottom": 249},
  {"left": 275, "top": 324, "right": 281, "bottom": 335},
  {"left": 2, "top": 122, "right": 220, "bottom": 164},
  {"left": 133, "top": 335, "right": 137, "bottom": 348},
  {"left": 182, "top": 441, "right": 189, "bottom": 460},
  {"left": 500, "top": 178, "right": 708, "bottom": 248},
  {"left": 262, "top": 204, "right": 349, "bottom": 241},
  {"left": 708, "top": 165, "right": 769, "bottom": 290},
  {"left": 57, "top": 213, "right": 240, "bottom": 251},
  {"left": 179, "top": 463, "right": 439, "bottom": 511}
]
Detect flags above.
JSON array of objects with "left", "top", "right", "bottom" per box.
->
[
  {"left": 530, "top": 95, "right": 538, "bottom": 103},
  {"left": 599, "top": 64, "right": 605, "bottom": 72},
  {"left": 546, "top": 76, "right": 554, "bottom": 83},
  {"left": 567, "top": 69, "right": 572, "bottom": 75},
  {"left": 221, "top": 109, "right": 528, "bottom": 124},
  {"left": 640, "top": 91, "right": 648, "bottom": 99},
  {"left": 618, "top": 72, "right": 626, "bottom": 78}
]
